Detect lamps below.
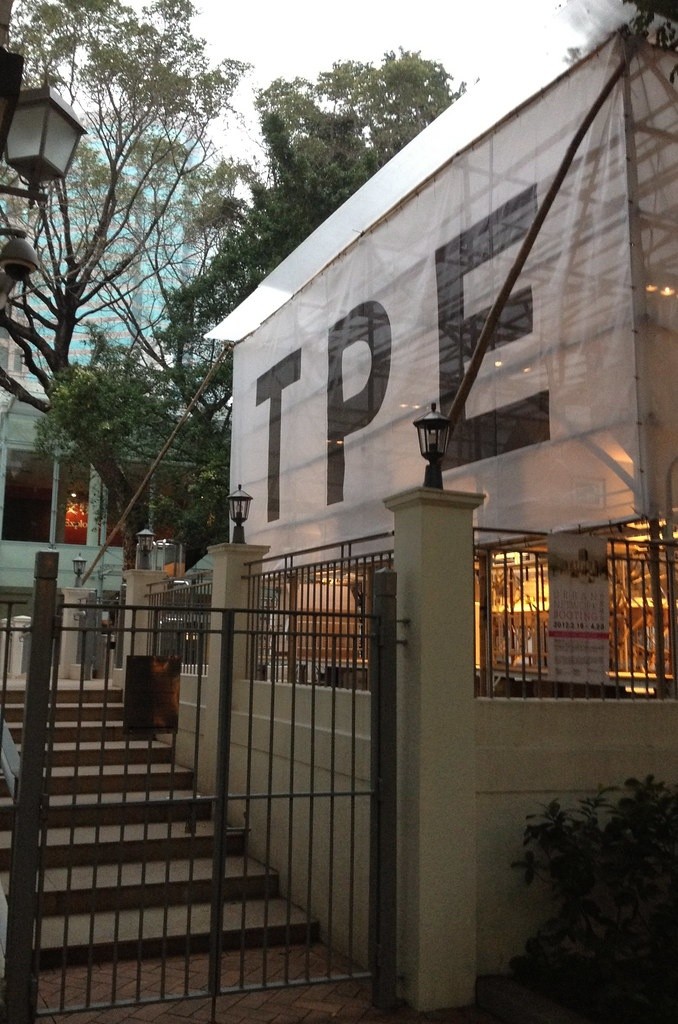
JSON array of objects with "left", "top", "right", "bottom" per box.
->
[
  {"left": 135, "top": 524, "right": 156, "bottom": 570},
  {"left": 72, "top": 552, "right": 87, "bottom": 588},
  {"left": 0, "top": 78, "right": 88, "bottom": 208},
  {"left": 412, "top": 402, "right": 452, "bottom": 491},
  {"left": 226, "top": 484, "right": 253, "bottom": 544}
]
